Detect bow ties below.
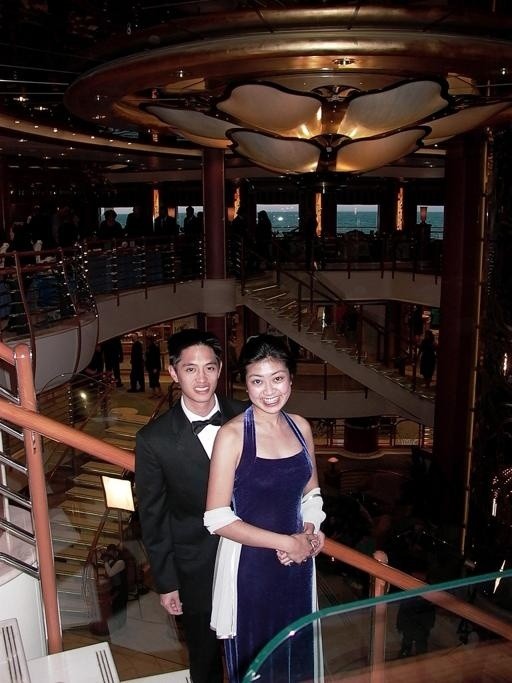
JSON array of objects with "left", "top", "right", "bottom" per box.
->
[{"left": 192, "top": 411, "right": 222, "bottom": 437}]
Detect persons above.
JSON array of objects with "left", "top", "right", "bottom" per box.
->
[
  {"left": 416, "top": 330, "right": 437, "bottom": 392},
  {"left": 202, "top": 333, "right": 327, "bottom": 683},
  {"left": 101, "top": 543, "right": 128, "bottom": 632},
  {"left": 397, "top": 596, "right": 436, "bottom": 657},
  {"left": 136, "top": 328, "right": 252, "bottom": 682},
  {"left": 410, "top": 304, "right": 424, "bottom": 337},
  {"left": 1, "top": 195, "right": 272, "bottom": 398}
]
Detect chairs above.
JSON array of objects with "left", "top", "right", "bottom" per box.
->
[{"left": 128, "top": 584, "right": 142, "bottom": 619}]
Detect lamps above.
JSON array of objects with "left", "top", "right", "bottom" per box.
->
[{"left": 139, "top": 75, "right": 511, "bottom": 175}]
